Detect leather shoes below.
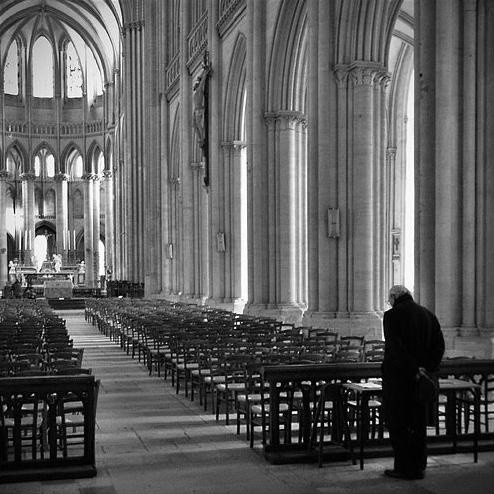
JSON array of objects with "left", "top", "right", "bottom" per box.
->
[{"left": 385, "top": 470, "right": 424, "bottom": 479}]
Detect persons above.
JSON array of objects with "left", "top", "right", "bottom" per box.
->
[
  {"left": 192, "top": 69, "right": 209, "bottom": 145},
  {"left": 378, "top": 284, "right": 446, "bottom": 481}
]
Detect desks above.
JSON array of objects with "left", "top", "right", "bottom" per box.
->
[{"left": 341, "top": 380, "right": 482, "bottom": 470}]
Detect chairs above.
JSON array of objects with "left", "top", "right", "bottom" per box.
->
[{"left": 0, "top": 299, "right": 102, "bottom": 459}]
